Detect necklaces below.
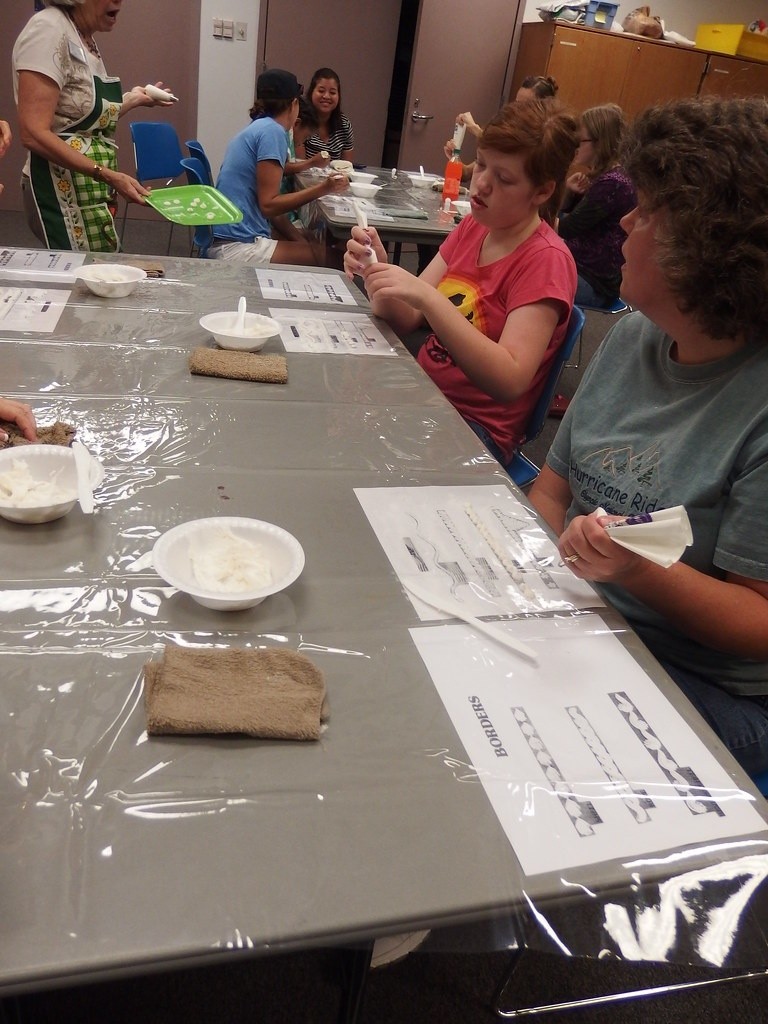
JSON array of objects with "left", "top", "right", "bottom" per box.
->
[{"left": 76, "top": 24, "right": 99, "bottom": 54}]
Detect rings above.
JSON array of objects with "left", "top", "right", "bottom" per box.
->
[{"left": 569, "top": 554, "right": 578, "bottom": 562}]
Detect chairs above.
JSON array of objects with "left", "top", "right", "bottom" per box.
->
[
  {"left": 121, "top": 122, "right": 191, "bottom": 256},
  {"left": 185, "top": 141, "right": 214, "bottom": 187},
  {"left": 558, "top": 297, "right": 630, "bottom": 368},
  {"left": 179, "top": 157, "right": 215, "bottom": 261},
  {"left": 504, "top": 306, "right": 585, "bottom": 490}
]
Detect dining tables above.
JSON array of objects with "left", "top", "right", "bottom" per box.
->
[{"left": 291, "top": 158, "right": 471, "bottom": 243}]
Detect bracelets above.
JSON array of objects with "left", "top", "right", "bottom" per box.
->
[{"left": 94, "top": 164, "right": 103, "bottom": 182}]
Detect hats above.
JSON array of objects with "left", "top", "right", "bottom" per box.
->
[{"left": 257, "top": 69, "right": 309, "bottom": 104}]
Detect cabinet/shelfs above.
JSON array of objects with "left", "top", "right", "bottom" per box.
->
[{"left": 510, "top": 23, "right": 768, "bottom": 127}]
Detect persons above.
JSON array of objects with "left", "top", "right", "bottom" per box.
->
[
  {"left": 296, "top": 68, "right": 355, "bottom": 162},
  {"left": 553, "top": 104, "right": 638, "bottom": 310},
  {"left": 344, "top": 96, "right": 580, "bottom": 470},
  {"left": 525, "top": 96, "right": 768, "bottom": 775},
  {"left": 416, "top": 74, "right": 557, "bottom": 275},
  {"left": 11, "top": 0, "right": 174, "bottom": 253},
  {"left": 205, "top": 68, "right": 340, "bottom": 269}
]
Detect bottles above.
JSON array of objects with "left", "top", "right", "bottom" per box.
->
[{"left": 441, "top": 149, "right": 463, "bottom": 206}]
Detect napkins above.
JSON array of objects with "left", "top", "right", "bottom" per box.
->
[
  {"left": 329, "top": 160, "right": 353, "bottom": 172},
  {"left": 377, "top": 209, "right": 429, "bottom": 220},
  {"left": 431, "top": 183, "right": 468, "bottom": 195}
]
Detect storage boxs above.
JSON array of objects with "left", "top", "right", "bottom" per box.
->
[
  {"left": 585, "top": 0, "right": 621, "bottom": 29},
  {"left": 695, "top": 20, "right": 768, "bottom": 63}
]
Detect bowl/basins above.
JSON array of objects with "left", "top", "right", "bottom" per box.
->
[
  {"left": 451, "top": 200, "right": 471, "bottom": 216},
  {"left": 0, "top": 445, "right": 104, "bottom": 525},
  {"left": 409, "top": 175, "right": 436, "bottom": 190},
  {"left": 350, "top": 172, "right": 375, "bottom": 185},
  {"left": 152, "top": 516, "right": 306, "bottom": 613},
  {"left": 349, "top": 182, "right": 382, "bottom": 198},
  {"left": 199, "top": 310, "right": 282, "bottom": 353},
  {"left": 75, "top": 264, "right": 146, "bottom": 298}
]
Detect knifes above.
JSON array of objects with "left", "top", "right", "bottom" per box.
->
[
  {"left": 71, "top": 441, "right": 94, "bottom": 515},
  {"left": 401, "top": 577, "right": 541, "bottom": 660}
]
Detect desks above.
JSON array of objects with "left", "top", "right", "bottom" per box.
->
[{"left": 0, "top": 246, "right": 768, "bottom": 999}]
